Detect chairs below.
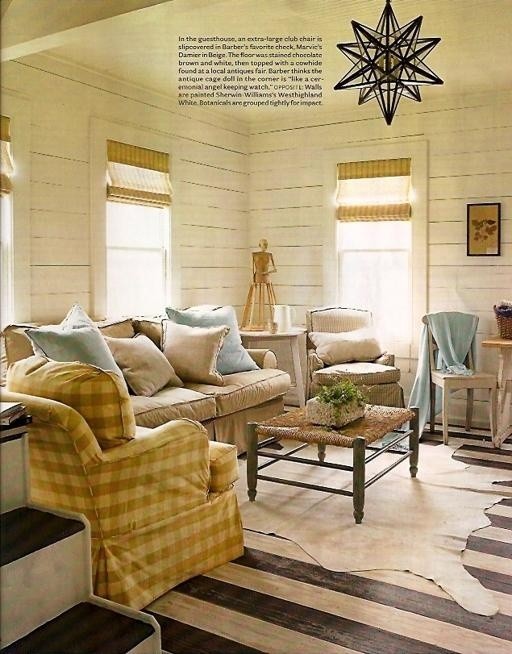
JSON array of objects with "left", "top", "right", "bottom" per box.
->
[
  {"left": 306, "top": 308, "right": 404, "bottom": 409},
  {"left": 422, "top": 312, "right": 497, "bottom": 445},
  {"left": 0, "top": 390, "right": 244, "bottom": 612}
]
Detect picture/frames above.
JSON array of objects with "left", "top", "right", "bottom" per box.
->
[{"left": 466, "top": 203, "right": 500, "bottom": 256}]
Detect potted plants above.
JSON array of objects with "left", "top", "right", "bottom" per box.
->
[{"left": 306, "top": 370, "right": 378, "bottom": 429}]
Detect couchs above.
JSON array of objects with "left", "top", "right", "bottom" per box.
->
[{"left": 0, "top": 313, "right": 291, "bottom": 458}]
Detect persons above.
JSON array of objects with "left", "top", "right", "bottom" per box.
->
[{"left": 251, "top": 238, "right": 278, "bottom": 283}]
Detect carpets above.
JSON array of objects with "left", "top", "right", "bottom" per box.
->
[{"left": 232, "top": 438, "right": 512, "bottom": 616}]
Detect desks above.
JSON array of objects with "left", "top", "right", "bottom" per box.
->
[
  {"left": 482, "top": 334, "right": 512, "bottom": 447},
  {"left": 239, "top": 327, "right": 306, "bottom": 408}
]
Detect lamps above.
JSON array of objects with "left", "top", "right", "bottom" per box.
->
[{"left": 334, "top": 0, "right": 444, "bottom": 125}]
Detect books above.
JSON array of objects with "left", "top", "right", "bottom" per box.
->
[
  {"left": 1, "top": 404, "right": 28, "bottom": 425},
  {"left": 1, "top": 398, "right": 24, "bottom": 417},
  {"left": 1, "top": 414, "right": 33, "bottom": 430}
]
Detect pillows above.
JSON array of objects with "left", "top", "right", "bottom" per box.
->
[
  {"left": 308, "top": 327, "right": 387, "bottom": 365},
  {"left": 0, "top": 306, "right": 260, "bottom": 397},
  {"left": 7, "top": 355, "right": 136, "bottom": 450}
]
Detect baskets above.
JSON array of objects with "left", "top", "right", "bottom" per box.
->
[{"left": 494, "top": 305, "right": 512, "bottom": 340}]
